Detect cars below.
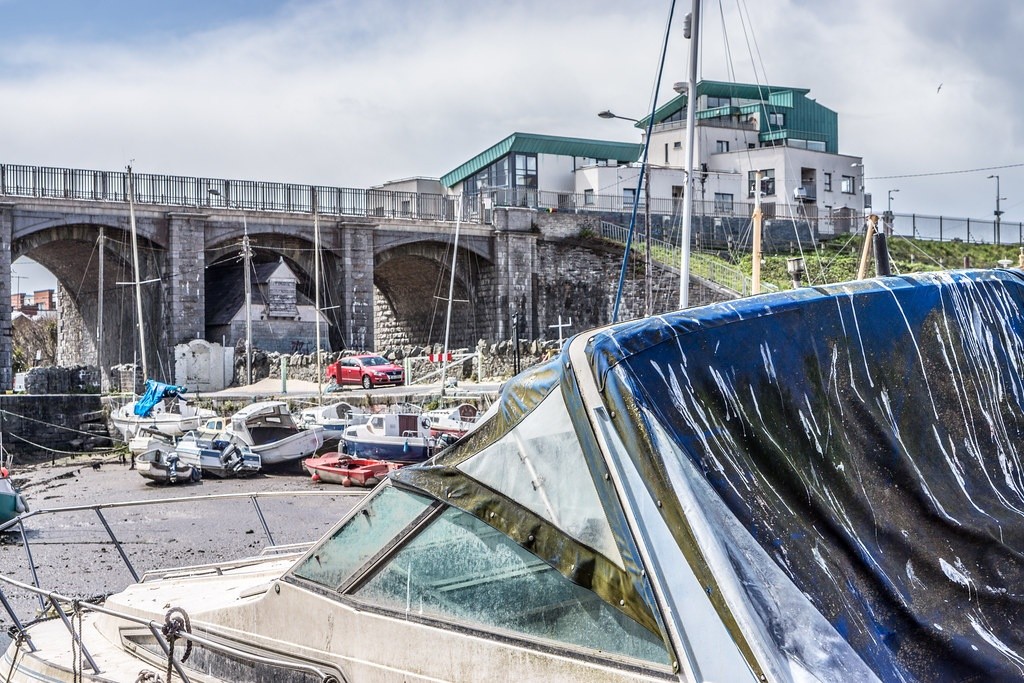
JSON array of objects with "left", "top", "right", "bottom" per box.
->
[{"left": 325, "top": 355, "right": 404, "bottom": 389}]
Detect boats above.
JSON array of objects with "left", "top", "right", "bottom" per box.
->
[
  {"left": 128, "top": 428, "right": 262, "bottom": 484},
  {"left": 1, "top": 265, "right": 1024, "bottom": 683},
  {"left": 302, "top": 453, "right": 405, "bottom": 487},
  {"left": 1, "top": 448, "right": 30, "bottom": 524}
]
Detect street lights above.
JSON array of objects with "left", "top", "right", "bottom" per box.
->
[
  {"left": 207, "top": 188, "right": 252, "bottom": 386},
  {"left": 987, "top": 174, "right": 1008, "bottom": 245},
  {"left": 597, "top": 110, "right": 652, "bottom": 316},
  {"left": 887, "top": 187, "right": 900, "bottom": 211}
]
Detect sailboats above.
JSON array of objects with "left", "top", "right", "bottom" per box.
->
[{"left": 105, "top": 164, "right": 492, "bottom": 459}]
